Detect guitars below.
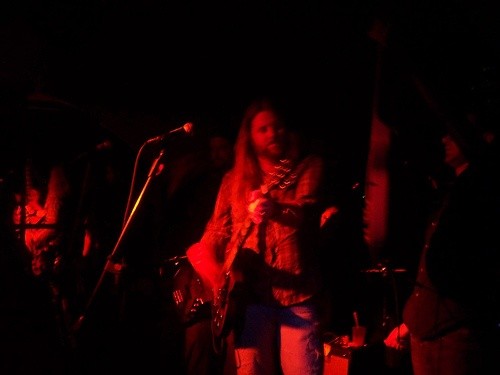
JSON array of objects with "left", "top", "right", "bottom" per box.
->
[{"left": 213, "top": 156, "right": 295, "bottom": 340}]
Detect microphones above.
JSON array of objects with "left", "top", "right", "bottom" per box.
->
[
  {"left": 146, "top": 120, "right": 194, "bottom": 143},
  {"left": 75, "top": 139, "right": 113, "bottom": 158}
]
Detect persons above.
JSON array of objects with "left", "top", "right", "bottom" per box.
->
[
  {"left": 380, "top": 110, "right": 500, "bottom": 374},
  {"left": 159, "top": 123, "right": 237, "bottom": 279},
  {"left": 189, "top": 98, "right": 329, "bottom": 375}
]
